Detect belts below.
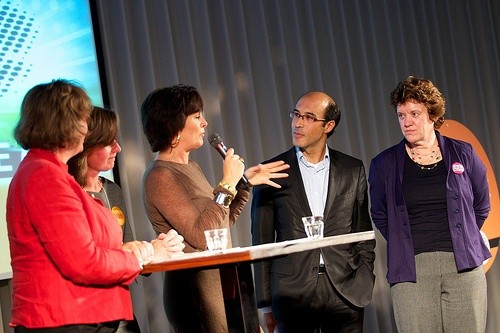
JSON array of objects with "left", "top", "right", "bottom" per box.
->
[{"left": 319, "top": 267, "right": 326, "bottom": 272}]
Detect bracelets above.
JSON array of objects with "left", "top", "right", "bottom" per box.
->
[{"left": 213, "top": 182, "right": 238, "bottom": 208}]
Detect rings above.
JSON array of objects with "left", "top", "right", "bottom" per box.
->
[{"left": 239, "top": 157, "right": 244, "bottom": 163}]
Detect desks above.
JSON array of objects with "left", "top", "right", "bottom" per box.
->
[{"left": 139, "top": 230, "right": 375, "bottom": 333}]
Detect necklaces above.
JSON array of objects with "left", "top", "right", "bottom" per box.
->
[
  {"left": 411, "top": 142, "right": 441, "bottom": 169},
  {"left": 81, "top": 180, "right": 112, "bottom": 212}
]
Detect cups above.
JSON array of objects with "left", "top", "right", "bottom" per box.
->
[
  {"left": 204, "top": 229, "right": 227, "bottom": 253},
  {"left": 302, "top": 216, "right": 324, "bottom": 239}
]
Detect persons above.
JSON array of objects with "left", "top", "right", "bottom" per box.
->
[
  {"left": 368, "top": 76, "right": 493, "bottom": 333},
  {"left": 67, "top": 106, "right": 185, "bottom": 333},
  {"left": 6, "top": 79, "right": 154, "bottom": 333},
  {"left": 141, "top": 83, "right": 289, "bottom": 333},
  {"left": 252, "top": 91, "right": 377, "bottom": 333}
]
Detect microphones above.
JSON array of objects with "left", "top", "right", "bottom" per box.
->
[{"left": 208, "top": 131, "right": 248, "bottom": 185}]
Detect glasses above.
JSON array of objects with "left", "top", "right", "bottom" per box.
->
[{"left": 289, "top": 111, "right": 327, "bottom": 123}]
